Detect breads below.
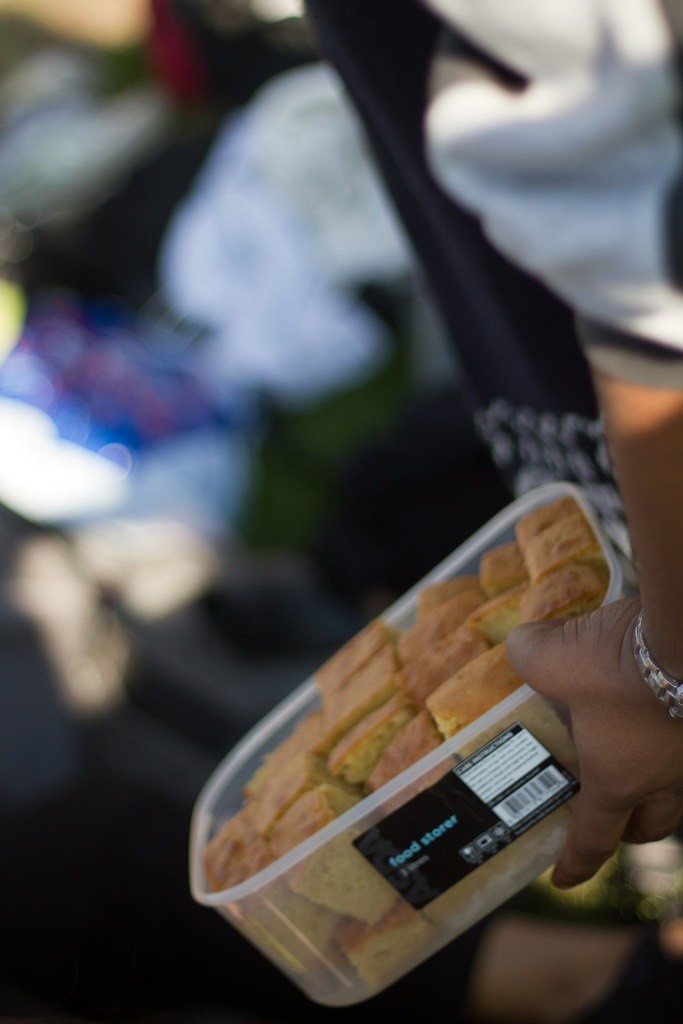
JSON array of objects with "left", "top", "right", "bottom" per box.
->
[{"left": 205, "top": 494, "right": 610, "bottom": 983}]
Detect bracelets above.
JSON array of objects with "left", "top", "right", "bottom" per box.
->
[{"left": 631, "top": 609, "right": 683, "bottom": 721}]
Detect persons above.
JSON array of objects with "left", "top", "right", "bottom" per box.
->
[{"left": 305, "top": 0, "right": 683, "bottom": 889}]
